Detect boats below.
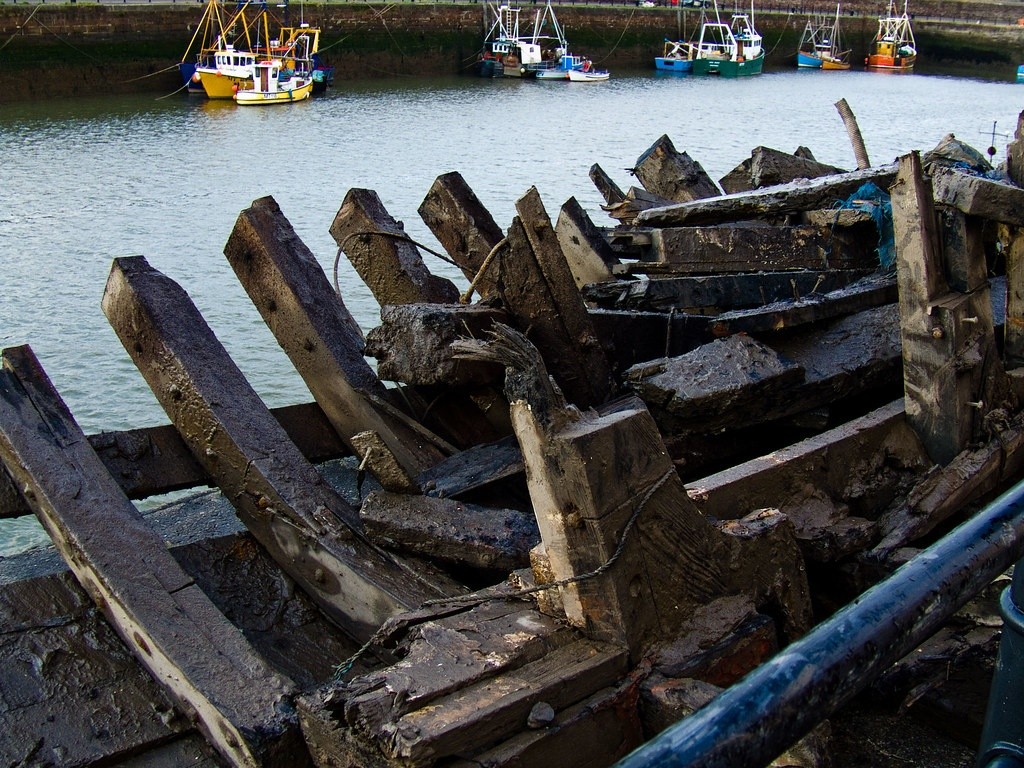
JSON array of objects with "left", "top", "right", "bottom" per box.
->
[
  {"left": 178, "top": 0, "right": 328, "bottom": 106},
  {"left": 652, "top": 0, "right": 766, "bottom": 76},
  {"left": 475, "top": 1, "right": 610, "bottom": 83},
  {"left": 796, "top": 4, "right": 852, "bottom": 70},
  {"left": 867, "top": 0, "right": 918, "bottom": 71}
]
